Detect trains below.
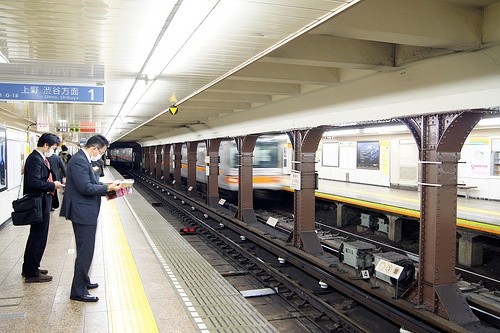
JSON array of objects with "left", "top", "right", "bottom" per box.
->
[{"left": 110, "top": 131, "right": 294, "bottom": 196}]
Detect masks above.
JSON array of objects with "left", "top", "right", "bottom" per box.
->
[
  {"left": 91, "top": 153, "right": 101, "bottom": 161},
  {"left": 43, "top": 147, "right": 53, "bottom": 157}
]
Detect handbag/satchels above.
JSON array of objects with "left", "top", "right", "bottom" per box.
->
[{"left": 11, "top": 193, "right": 44, "bottom": 225}]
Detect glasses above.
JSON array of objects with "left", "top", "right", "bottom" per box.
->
[{"left": 96, "top": 146, "right": 105, "bottom": 155}]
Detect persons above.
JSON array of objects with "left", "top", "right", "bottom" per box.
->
[
  {"left": 11, "top": 132, "right": 67, "bottom": 284},
  {"left": 45, "top": 144, "right": 72, "bottom": 212},
  {"left": 65, "top": 133, "right": 123, "bottom": 303}
]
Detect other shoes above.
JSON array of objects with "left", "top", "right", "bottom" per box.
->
[
  {"left": 25, "top": 274, "right": 53, "bottom": 282},
  {"left": 39, "top": 269, "right": 49, "bottom": 274},
  {"left": 87, "top": 282, "right": 98, "bottom": 288},
  {"left": 70, "top": 293, "right": 99, "bottom": 301}
]
